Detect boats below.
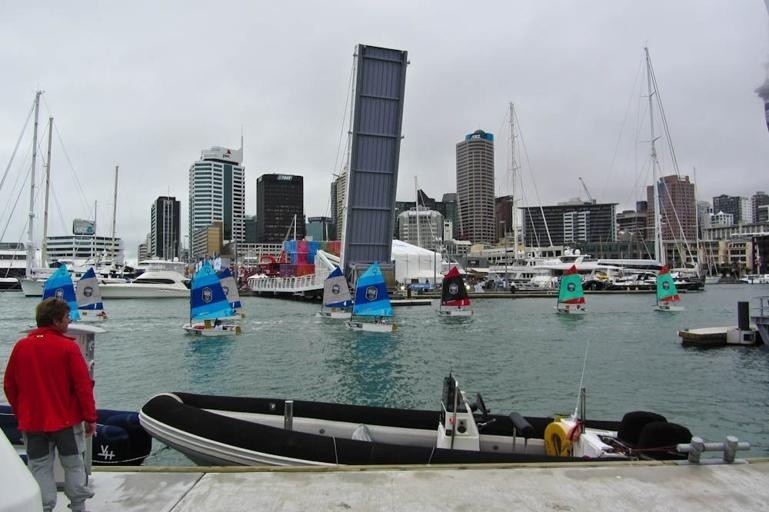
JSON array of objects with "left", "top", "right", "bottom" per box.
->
[
  {"left": 678, "top": 324, "right": 759, "bottom": 347},
  {"left": 0, "top": 403, "right": 152, "bottom": 467},
  {"left": 137, "top": 368, "right": 695, "bottom": 469}
]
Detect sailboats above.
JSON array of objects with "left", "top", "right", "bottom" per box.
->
[
  {"left": 36, "top": 261, "right": 109, "bottom": 324},
  {"left": 181, "top": 258, "right": 247, "bottom": 337},
  {"left": 316, "top": 259, "right": 400, "bottom": 334},
  {"left": 553, "top": 264, "right": 587, "bottom": 315},
  {"left": 437, "top": 266, "right": 474, "bottom": 318},
  {"left": 652, "top": 266, "right": 684, "bottom": 313}
]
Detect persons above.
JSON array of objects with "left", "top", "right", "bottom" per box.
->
[{"left": 3, "top": 297, "right": 97, "bottom": 512}]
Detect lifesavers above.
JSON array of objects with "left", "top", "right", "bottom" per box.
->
[{"left": 544, "top": 421, "right": 571, "bottom": 459}]
